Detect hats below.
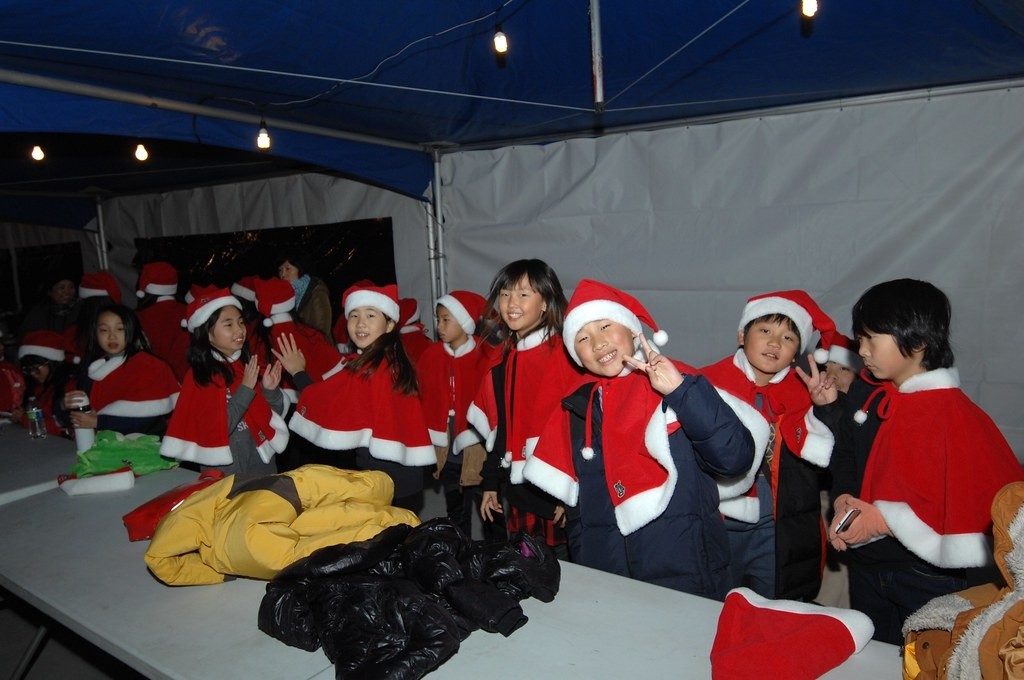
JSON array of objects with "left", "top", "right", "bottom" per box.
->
[
  {"left": 344, "top": 284, "right": 400, "bottom": 324},
  {"left": 18, "top": 329, "right": 81, "bottom": 364},
  {"left": 815, "top": 334, "right": 861, "bottom": 373},
  {"left": 562, "top": 279, "right": 669, "bottom": 369},
  {"left": 136, "top": 262, "right": 178, "bottom": 298},
  {"left": 181, "top": 285, "right": 243, "bottom": 332},
  {"left": 433, "top": 289, "right": 503, "bottom": 338},
  {"left": 737, "top": 289, "right": 835, "bottom": 364},
  {"left": 397, "top": 298, "right": 420, "bottom": 329},
  {"left": 709, "top": 587, "right": 876, "bottom": 680},
  {"left": 57, "top": 467, "right": 138, "bottom": 496},
  {"left": 230, "top": 275, "right": 260, "bottom": 301},
  {"left": 79, "top": 270, "right": 120, "bottom": 303},
  {"left": 255, "top": 277, "right": 296, "bottom": 327}
]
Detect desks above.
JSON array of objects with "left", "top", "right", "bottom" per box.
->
[{"left": 0, "top": 419, "right": 911, "bottom": 680}]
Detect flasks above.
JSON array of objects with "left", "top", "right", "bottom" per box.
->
[{"left": 70, "top": 395, "right": 94, "bottom": 452}]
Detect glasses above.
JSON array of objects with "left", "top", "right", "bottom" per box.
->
[{"left": 22, "top": 361, "right": 49, "bottom": 375}]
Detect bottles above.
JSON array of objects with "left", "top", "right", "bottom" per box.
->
[{"left": 26, "top": 396, "right": 47, "bottom": 439}]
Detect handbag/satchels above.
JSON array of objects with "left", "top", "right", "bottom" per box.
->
[
  {"left": 123, "top": 469, "right": 225, "bottom": 542},
  {"left": 71, "top": 429, "right": 181, "bottom": 477}
]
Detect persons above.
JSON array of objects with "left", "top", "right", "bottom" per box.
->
[
  {"left": 0, "top": 254, "right": 882, "bottom": 603},
  {"left": 822, "top": 276, "right": 1024, "bottom": 646}
]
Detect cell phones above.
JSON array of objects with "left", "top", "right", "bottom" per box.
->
[{"left": 834, "top": 509, "right": 862, "bottom": 534}]
